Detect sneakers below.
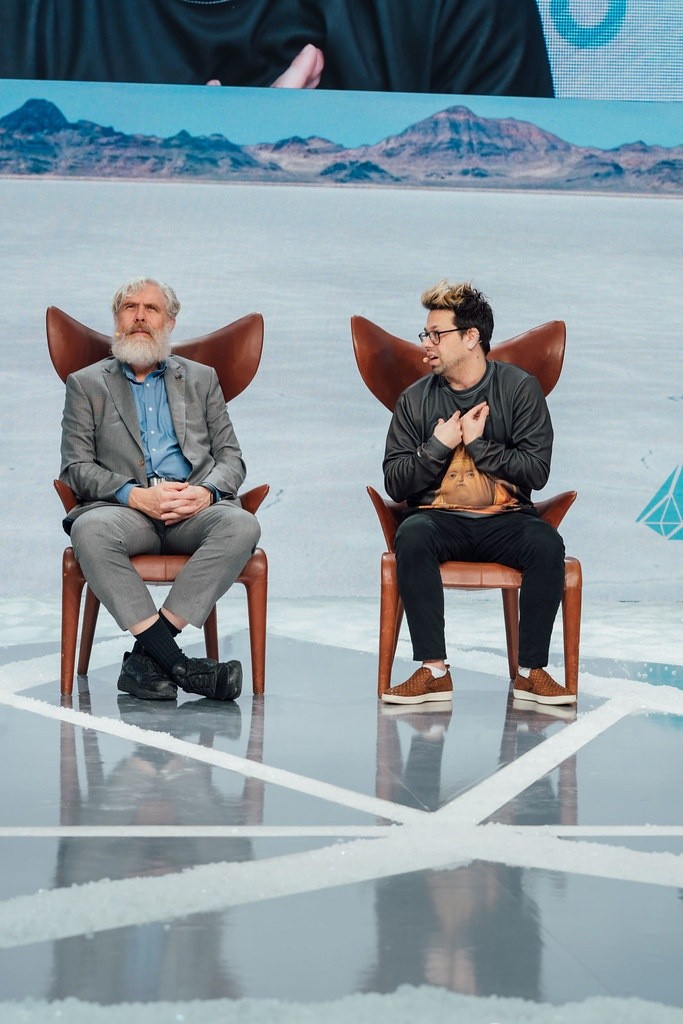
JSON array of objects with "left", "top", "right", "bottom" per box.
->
[
  {"left": 513, "top": 668, "right": 576, "bottom": 704},
  {"left": 383, "top": 664, "right": 453, "bottom": 704}
]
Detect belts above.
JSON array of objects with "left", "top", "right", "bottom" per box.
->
[{"left": 148, "top": 477, "right": 181, "bottom": 487}]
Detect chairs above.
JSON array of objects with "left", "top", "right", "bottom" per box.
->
[
  {"left": 351, "top": 314, "right": 584, "bottom": 698},
  {"left": 46, "top": 305, "right": 269, "bottom": 696}
]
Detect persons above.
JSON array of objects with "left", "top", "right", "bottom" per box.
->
[
  {"left": 60, "top": 278, "right": 261, "bottom": 703},
  {"left": 382, "top": 284, "right": 577, "bottom": 705}
]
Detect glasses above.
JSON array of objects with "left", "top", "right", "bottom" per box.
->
[{"left": 418, "top": 328, "right": 468, "bottom": 345}]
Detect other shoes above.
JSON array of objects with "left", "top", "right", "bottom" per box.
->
[
  {"left": 116, "top": 652, "right": 177, "bottom": 700},
  {"left": 175, "top": 657, "right": 243, "bottom": 703}
]
